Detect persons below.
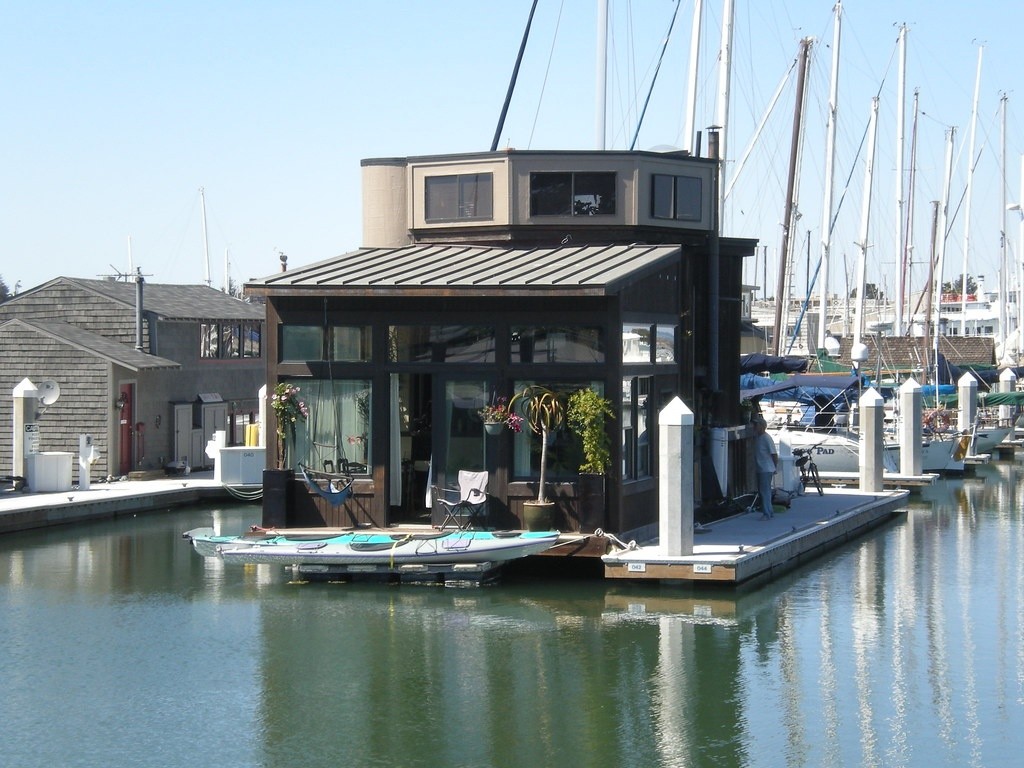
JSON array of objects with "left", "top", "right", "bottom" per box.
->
[
  {"left": 752, "top": 416, "right": 779, "bottom": 522},
  {"left": 410, "top": 430, "right": 433, "bottom": 513}
]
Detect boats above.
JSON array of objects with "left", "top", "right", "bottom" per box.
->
[{"left": 177, "top": 518, "right": 564, "bottom": 581}]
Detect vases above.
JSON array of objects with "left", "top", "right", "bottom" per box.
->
[{"left": 484, "top": 422, "right": 503, "bottom": 434}]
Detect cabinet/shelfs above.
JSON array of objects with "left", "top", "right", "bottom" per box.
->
[
  {"left": 192, "top": 429, "right": 203, "bottom": 470},
  {"left": 196, "top": 401, "right": 228, "bottom": 469},
  {"left": 169, "top": 402, "right": 192, "bottom": 470},
  {"left": 27, "top": 452, "right": 75, "bottom": 491},
  {"left": 214, "top": 447, "right": 266, "bottom": 484}
]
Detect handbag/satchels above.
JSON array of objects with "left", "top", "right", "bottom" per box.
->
[{"left": 772, "top": 488, "right": 794, "bottom": 506}]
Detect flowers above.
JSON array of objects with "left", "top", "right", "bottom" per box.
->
[
  {"left": 271, "top": 383, "right": 308, "bottom": 470},
  {"left": 478, "top": 397, "right": 524, "bottom": 432}
]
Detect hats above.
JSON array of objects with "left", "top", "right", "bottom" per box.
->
[{"left": 752, "top": 418, "right": 767, "bottom": 428}]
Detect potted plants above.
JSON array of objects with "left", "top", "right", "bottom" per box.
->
[
  {"left": 567, "top": 388, "right": 617, "bottom": 532},
  {"left": 262, "top": 469, "right": 296, "bottom": 529},
  {"left": 508, "top": 385, "right": 564, "bottom": 532}
]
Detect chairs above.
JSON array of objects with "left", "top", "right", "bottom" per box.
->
[
  {"left": 430, "top": 470, "right": 490, "bottom": 532},
  {"left": 323, "top": 458, "right": 349, "bottom": 473}
]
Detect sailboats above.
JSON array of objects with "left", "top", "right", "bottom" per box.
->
[{"left": 580, "top": 0, "right": 1023, "bottom": 489}]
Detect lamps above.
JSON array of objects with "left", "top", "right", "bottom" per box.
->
[{"left": 116, "top": 391, "right": 129, "bottom": 408}]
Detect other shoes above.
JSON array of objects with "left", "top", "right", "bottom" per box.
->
[
  {"left": 760, "top": 515, "right": 775, "bottom": 520},
  {"left": 694, "top": 523, "right": 712, "bottom": 533}
]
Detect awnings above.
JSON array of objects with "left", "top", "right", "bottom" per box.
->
[{"left": 244, "top": 244, "right": 682, "bottom": 296}]
